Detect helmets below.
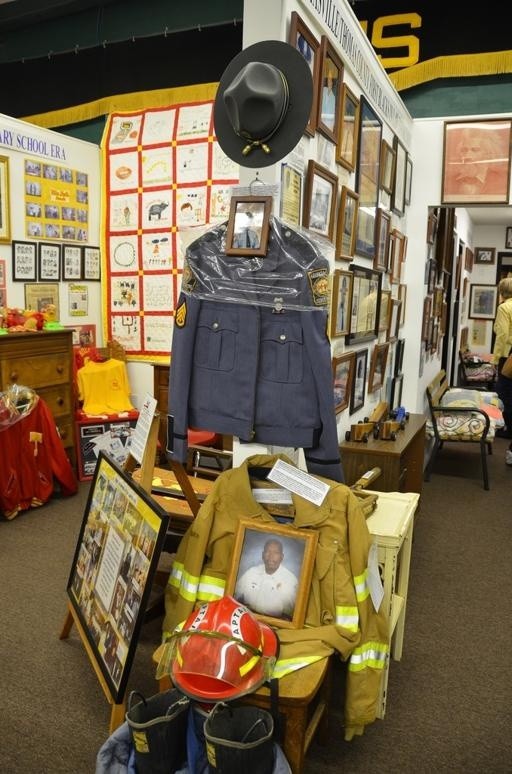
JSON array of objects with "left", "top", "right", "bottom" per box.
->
[{"left": 152, "top": 596, "right": 278, "bottom": 704}]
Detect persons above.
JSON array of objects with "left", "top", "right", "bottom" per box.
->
[
  {"left": 236, "top": 210, "right": 262, "bottom": 252},
  {"left": 237, "top": 536, "right": 299, "bottom": 619},
  {"left": 488, "top": 277, "right": 511, "bottom": 464},
  {"left": 353, "top": 360, "right": 366, "bottom": 406},
  {"left": 336, "top": 275, "right": 349, "bottom": 333}
]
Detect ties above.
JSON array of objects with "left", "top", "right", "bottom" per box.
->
[{"left": 247, "top": 230, "right": 250, "bottom": 247}]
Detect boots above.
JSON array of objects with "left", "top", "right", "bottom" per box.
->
[
  {"left": 125, "top": 689, "right": 190, "bottom": 774},
  {"left": 204, "top": 701, "right": 274, "bottom": 774}
]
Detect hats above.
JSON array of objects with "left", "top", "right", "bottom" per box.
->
[{"left": 214, "top": 41, "right": 313, "bottom": 168}]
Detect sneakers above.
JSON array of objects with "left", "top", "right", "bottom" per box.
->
[{"left": 505, "top": 450, "right": 512, "bottom": 464}]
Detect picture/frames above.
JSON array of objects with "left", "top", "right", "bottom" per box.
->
[
  {"left": 0, "top": 155, "right": 12, "bottom": 246},
  {"left": 421, "top": 205, "right": 512, "bottom": 355},
  {"left": 222, "top": 522, "right": 321, "bottom": 629},
  {"left": 10, "top": 239, "right": 101, "bottom": 282},
  {"left": 440, "top": 115, "right": 511, "bottom": 205},
  {"left": 224, "top": 193, "right": 273, "bottom": 258}
]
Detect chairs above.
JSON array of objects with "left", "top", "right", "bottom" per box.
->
[
  {"left": 159, "top": 469, "right": 338, "bottom": 770},
  {"left": 425, "top": 346, "right": 505, "bottom": 491}
]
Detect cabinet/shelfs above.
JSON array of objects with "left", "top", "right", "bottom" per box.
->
[
  {"left": 1, "top": 327, "right": 80, "bottom": 490},
  {"left": 339, "top": 408, "right": 429, "bottom": 517}
]
